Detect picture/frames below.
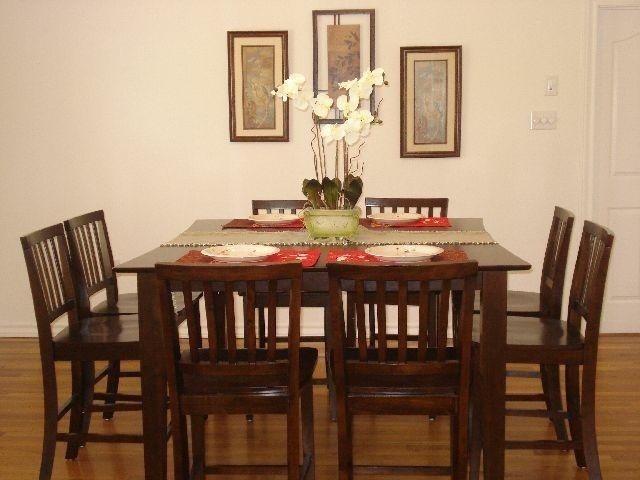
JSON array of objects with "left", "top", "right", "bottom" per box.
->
[
  {"left": 312, "top": 6, "right": 378, "bottom": 128},
  {"left": 227, "top": 27, "right": 291, "bottom": 144},
  {"left": 398, "top": 43, "right": 464, "bottom": 159}
]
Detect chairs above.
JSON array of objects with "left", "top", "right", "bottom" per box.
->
[
  {"left": 155, "top": 259, "right": 318, "bottom": 480},
  {"left": 475, "top": 203, "right": 576, "bottom": 452},
  {"left": 237, "top": 199, "right": 338, "bottom": 425},
  {"left": 325, "top": 259, "right": 479, "bottom": 480},
  {"left": 365, "top": 197, "right": 447, "bottom": 419},
  {"left": 20, "top": 224, "right": 172, "bottom": 480},
  {"left": 64, "top": 209, "right": 203, "bottom": 445},
  {"left": 469, "top": 219, "right": 614, "bottom": 480}
]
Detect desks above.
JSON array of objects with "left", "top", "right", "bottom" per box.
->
[{"left": 112, "top": 216, "right": 531, "bottom": 478}]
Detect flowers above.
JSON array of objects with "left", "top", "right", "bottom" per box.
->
[{"left": 270, "top": 66, "right": 398, "bottom": 212}]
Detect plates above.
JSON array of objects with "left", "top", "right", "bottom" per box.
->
[
  {"left": 367, "top": 211, "right": 424, "bottom": 223},
  {"left": 201, "top": 244, "right": 281, "bottom": 262},
  {"left": 248, "top": 213, "right": 301, "bottom": 224},
  {"left": 364, "top": 244, "right": 445, "bottom": 263}
]
